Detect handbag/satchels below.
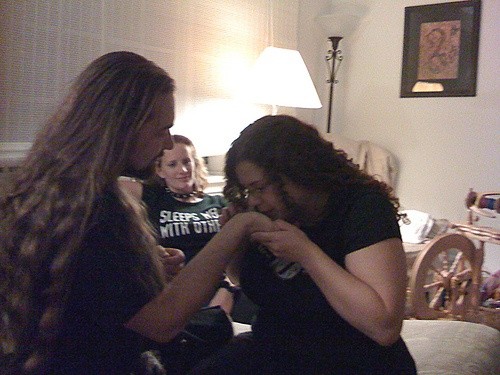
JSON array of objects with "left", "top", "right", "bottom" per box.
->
[{"left": 397, "top": 209, "right": 435, "bottom": 244}]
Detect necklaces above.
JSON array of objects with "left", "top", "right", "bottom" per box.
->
[{"left": 166, "top": 187, "right": 197, "bottom": 198}]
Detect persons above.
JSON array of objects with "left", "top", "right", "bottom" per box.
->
[
  {"left": 0, "top": 51, "right": 282, "bottom": 375},
  {"left": 148, "top": 135, "right": 243, "bottom": 317},
  {"left": 218, "top": 115, "right": 417, "bottom": 375}
]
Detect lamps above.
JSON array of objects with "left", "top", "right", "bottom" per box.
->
[
  {"left": 239, "top": 46, "right": 322, "bottom": 117},
  {"left": 313, "top": 13, "right": 361, "bottom": 135}
]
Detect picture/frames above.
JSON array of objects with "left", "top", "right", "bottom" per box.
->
[{"left": 399, "top": 0, "right": 481, "bottom": 98}]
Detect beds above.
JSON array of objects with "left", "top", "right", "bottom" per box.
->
[{"left": 229, "top": 319, "right": 500, "bottom": 374}]
